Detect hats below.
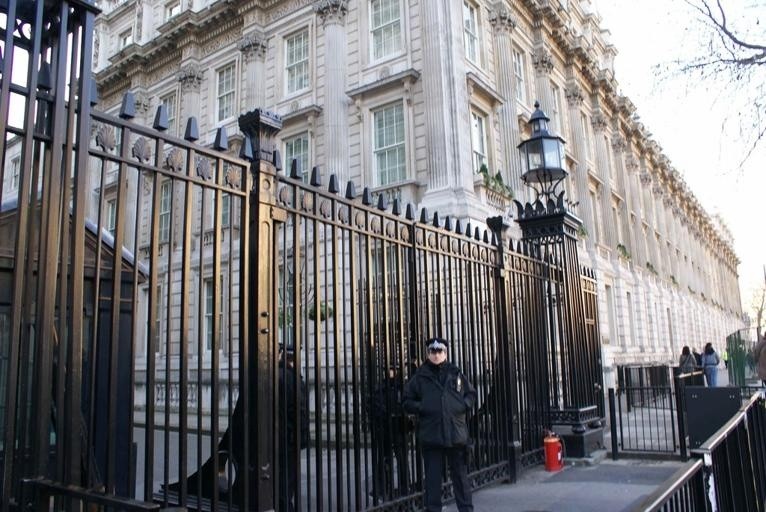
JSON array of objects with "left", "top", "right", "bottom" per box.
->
[
  {"left": 278, "top": 343, "right": 302, "bottom": 354},
  {"left": 426, "top": 338, "right": 448, "bottom": 350}
]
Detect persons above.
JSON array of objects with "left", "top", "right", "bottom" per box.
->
[
  {"left": 278, "top": 344, "right": 308, "bottom": 511},
  {"left": 370, "top": 338, "right": 478, "bottom": 512},
  {"left": 701, "top": 343, "right": 720, "bottom": 386},
  {"left": 746, "top": 348, "right": 756, "bottom": 372},
  {"left": 692, "top": 347, "right": 701, "bottom": 366},
  {"left": 679, "top": 345, "right": 697, "bottom": 372},
  {"left": 754, "top": 331, "right": 766, "bottom": 386},
  {"left": 723, "top": 348, "right": 728, "bottom": 369}
]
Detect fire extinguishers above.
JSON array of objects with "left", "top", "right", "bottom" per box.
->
[{"left": 543, "top": 428, "right": 565, "bottom": 472}]
